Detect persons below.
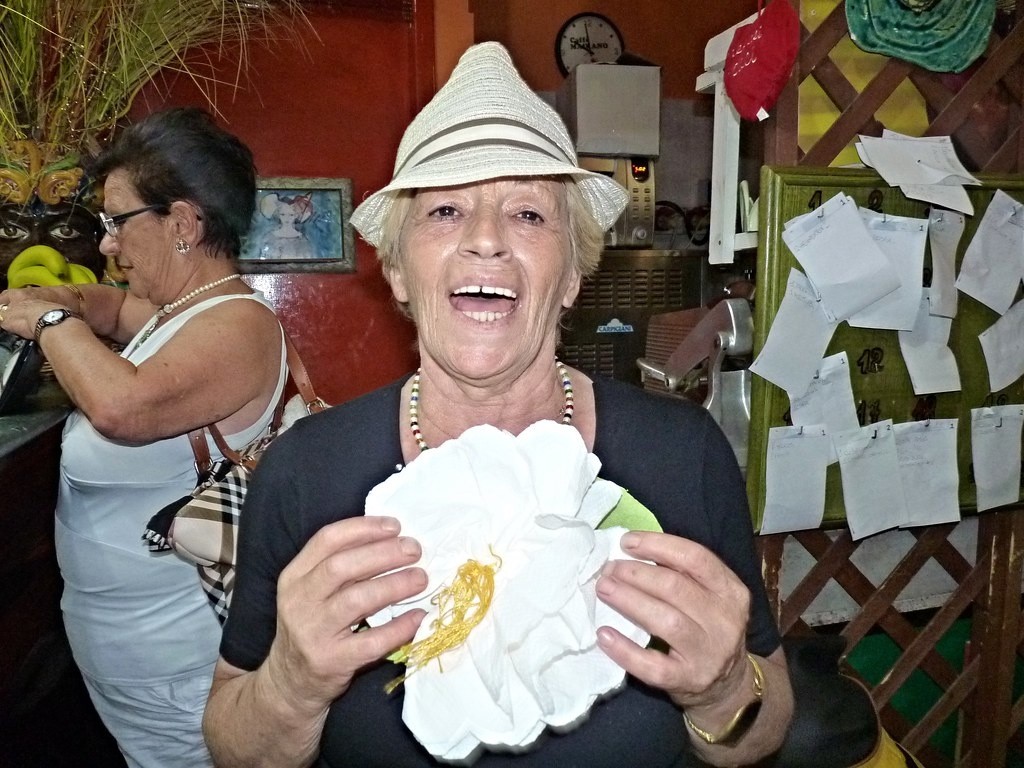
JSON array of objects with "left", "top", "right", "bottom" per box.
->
[
  {"left": 0, "top": 110, "right": 289, "bottom": 768},
  {"left": 203, "top": 40, "right": 796, "bottom": 768},
  {"left": 0, "top": 142, "right": 125, "bottom": 290}
]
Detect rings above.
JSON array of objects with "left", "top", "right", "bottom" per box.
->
[{"left": 0, "top": 304, "right": 7, "bottom": 322}]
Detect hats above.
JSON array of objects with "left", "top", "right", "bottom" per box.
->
[{"left": 346, "top": 41, "right": 631, "bottom": 248}]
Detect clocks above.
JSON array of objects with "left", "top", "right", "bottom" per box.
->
[{"left": 555, "top": 12, "right": 626, "bottom": 80}]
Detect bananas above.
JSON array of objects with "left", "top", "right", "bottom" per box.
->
[{"left": 7, "top": 244, "right": 98, "bottom": 289}]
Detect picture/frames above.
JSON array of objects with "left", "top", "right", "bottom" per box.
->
[{"left": 231, "top": 175, "right": 359, "bottom": 274}]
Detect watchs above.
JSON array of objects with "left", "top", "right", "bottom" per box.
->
[{"left": 35, "top": 308, "right": 83, "bottom": 343}]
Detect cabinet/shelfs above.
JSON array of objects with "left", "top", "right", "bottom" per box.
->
[{"left": 0, "top": 383, "right": 79, "bottom": 712}]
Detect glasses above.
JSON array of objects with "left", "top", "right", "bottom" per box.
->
[{"left": 99, "top": 205, "right": 204, "bottom": 239}]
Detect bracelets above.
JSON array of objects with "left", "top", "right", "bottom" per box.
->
[
  {"left": 682, "top": 655, "right": 765, "bottom": 745},
  {"left": 64, "top": 283, "right": 83, "bottom": 312}
]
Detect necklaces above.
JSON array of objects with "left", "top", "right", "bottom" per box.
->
[
  {"left": 135, "top": 274, "right": 240, "bottom": 348},
  {"left": 421, "top": 405, "right": 565, "bottom": 438},
  {"left": 409, "top": 355, "right": 575, "bottom": 452}
]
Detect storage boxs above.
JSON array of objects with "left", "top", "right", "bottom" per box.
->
[{"left": 554, "top": 62, "right": 661, "bottom": 158}]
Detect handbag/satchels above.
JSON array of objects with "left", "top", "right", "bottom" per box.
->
[{"left": 144, "top": 296, "right": 336, "bottom": 631}]
